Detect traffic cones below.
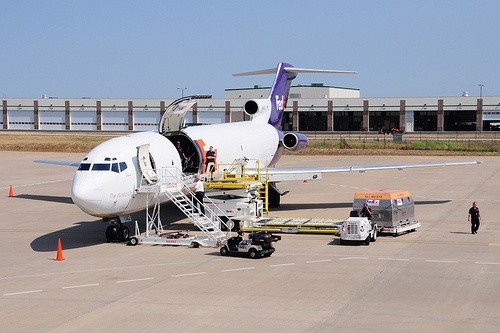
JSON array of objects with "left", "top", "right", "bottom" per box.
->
[
  {"left": 52, "top": 238, "right": 65, "bottom": 261},
  {"left": 7, "top": 183, "right": 15, "bottom": 198}
]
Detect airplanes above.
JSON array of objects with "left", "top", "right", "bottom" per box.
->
[{"left": 32, "top": 61, "right": 485, "bottom": 243}]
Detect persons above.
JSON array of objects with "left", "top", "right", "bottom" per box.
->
[
  {"left": 468, "top": 202, "right": 481, "bottom": 234},
  {"left": 204, "top": 146, "right": 216, "bottom": 182},
  {"left": 360, "top": 203, "right": 372, "bottom": 220},
  {"left": 175, "top": 141, "right": 188, "bottom": 159},
  {"left": 187, "top": 176, "right": 204, "bottom": 216}
]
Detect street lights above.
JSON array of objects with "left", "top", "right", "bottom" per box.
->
[
  {"left": 478, "top": 85, "right": 485, "bottom": 134},
  {"left": 177, "top": 87, "right": 188, "bottom": 97}
]
